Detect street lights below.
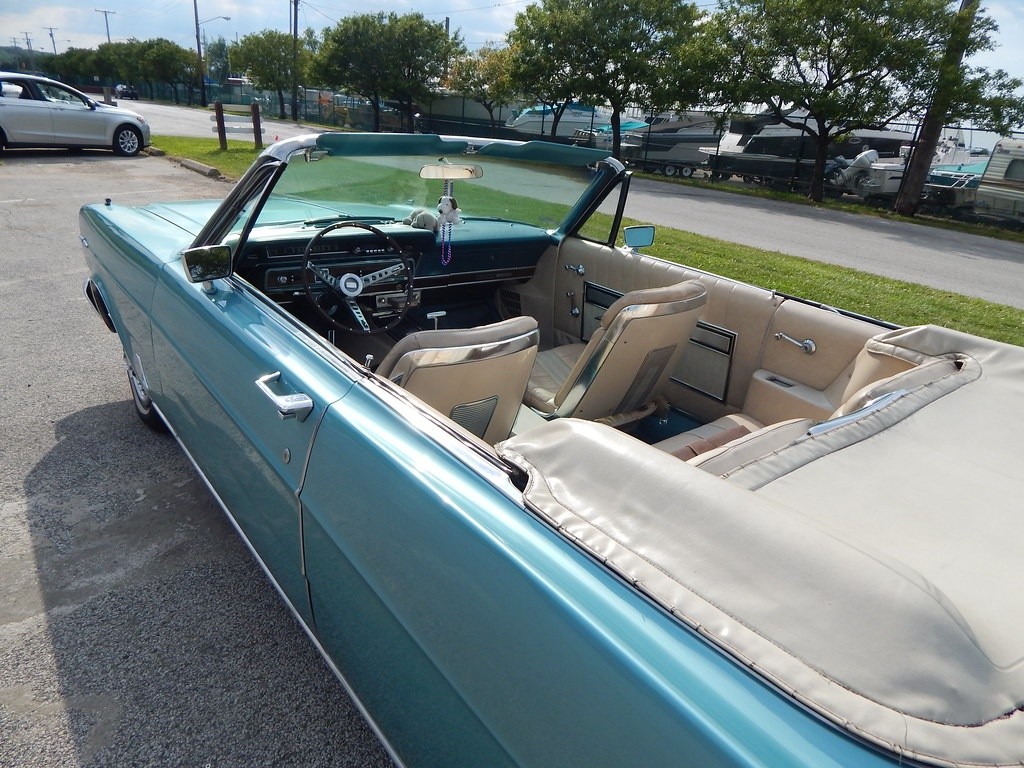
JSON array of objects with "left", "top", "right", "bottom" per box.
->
[
  {"left": 196, "top": 16, "right": 231, "bottom": 108},
  {"left": 53, "top": 39, "right": 71, "bottom": 56},
  {"left": 28, "top": 46, "right": 44, "bottom": 73}
]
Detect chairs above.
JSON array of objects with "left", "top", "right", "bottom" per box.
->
[
  {"left": 521, "top": 278, "right": 709, "bottom": 433},
  {"left": 375, "top": 315, "right": 540, "bottom": 447}
]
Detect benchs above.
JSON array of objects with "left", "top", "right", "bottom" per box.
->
[{"left": 650, "top": 340, "right": 982, "bottom": 491}]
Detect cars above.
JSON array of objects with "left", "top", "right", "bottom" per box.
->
[
  {"left": 0, "top": 72, "right": 152, "bottom": 158},
  {"left": 75, "top": 130, "right": 1024, "bottom": 767},
  {"left": 115, "top": 84, "right": 138, "bottom": 100}
]
